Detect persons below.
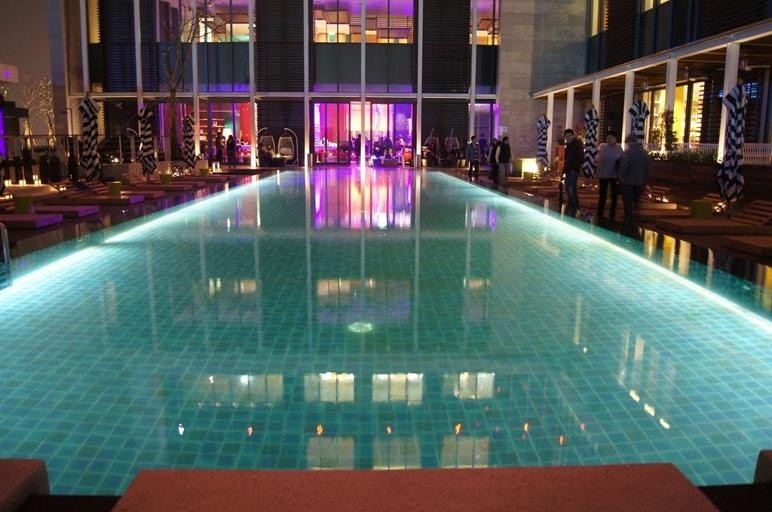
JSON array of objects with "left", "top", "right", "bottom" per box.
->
[
  {"left": 465, "top": 136, "right": 479, "bottom": 172},
  {"left": 215, "top": 132, "right": 223, "bottom": 169},
  {"left": 227, "top": 135, "right": 237, "bottom": 168},
  {"left": 594, "top": 134, "right": 623, "bottom": 218},
  {"left": 341, "top": 131, "right": 409, "bottom": 168},
  {"left": 616, "top": 133, "right": 651, "bottom": 222},
  {"left": 562, "top": 129, "right": 585, "bottom": 210},
  {"left": 495, "top": 136, "right": 513, "bottom": 186},
  {"left": 477, "top": 133, "right": 502, "bottom": 189}
]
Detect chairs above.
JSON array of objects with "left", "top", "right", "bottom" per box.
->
[
  {"left": 577, "top": 184, "right": 771, "bottom": 236},
  {"left": 44, "top": 171, "right": 193, "bottom": 206}
]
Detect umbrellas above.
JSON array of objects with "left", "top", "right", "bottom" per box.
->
[
  {"left": 535, "top": 115, "right": 550, "bottom": 173},
  {"left": 580, "top": 106, "right": 600, "bottom": 181},
  {"left": 181, "top": 116, "right": 199, "bottom": 169},
  {"left": 717, "top": 79, "right": 747, "bottom": 203},
  {"left": 624, "top": 99, "right": 649, "bottom": 146},
  {"left": 76, "top": 94, "right": 104, "bottom": 192},
  {"left": 135, "top": 106, "right": 158, "bottom": 179}
]
